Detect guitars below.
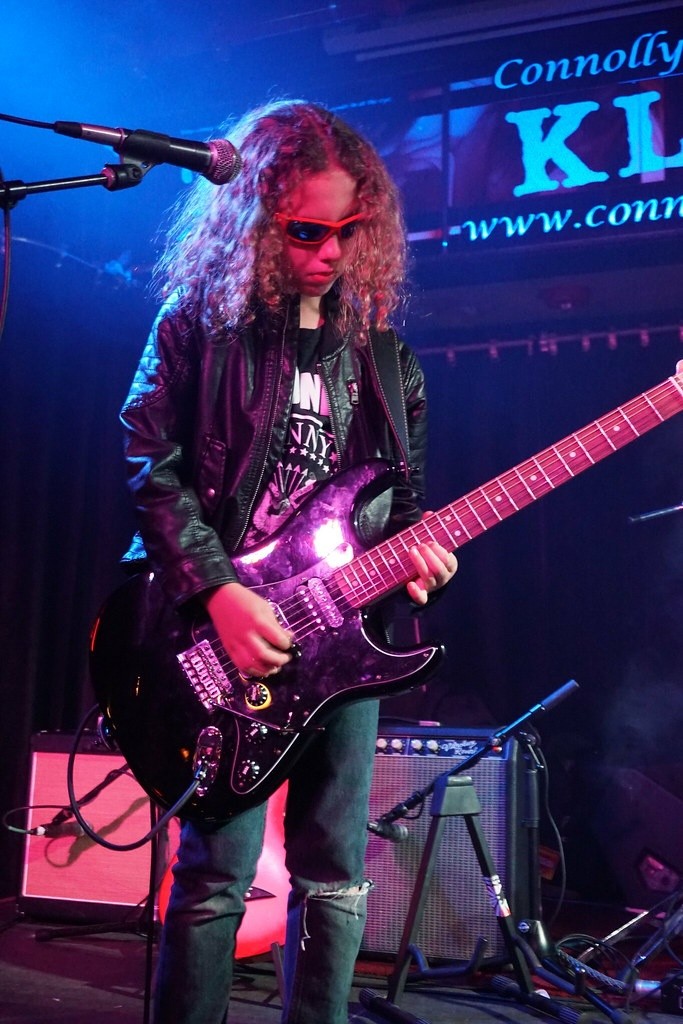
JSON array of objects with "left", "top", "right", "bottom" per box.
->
[{"left": 77, "top": 355, "right": 683, "bottom": 835}]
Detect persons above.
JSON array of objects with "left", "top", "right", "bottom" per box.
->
[{"left": 117, "top": 98, "right": 459, "bottom": 1024}]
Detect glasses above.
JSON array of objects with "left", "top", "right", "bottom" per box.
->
[{"left": 272, "top": 212, "right": 369, "bottom": 245}]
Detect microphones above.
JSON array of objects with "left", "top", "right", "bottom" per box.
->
[
  {"left": 30, "top": 821, "right": 92, "bottom": 837},
  {"left": 51, "top": 120, "right": 241, "bottom": 184},
  {"left": 364, "top": 821, "right": 409, "bottom": 843}
]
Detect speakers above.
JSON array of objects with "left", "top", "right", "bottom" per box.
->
[
  {"left": 15, "top": 734, "right": 184, "bottom": 921},
  {"left": 355, "top": 720, "right": 543, "bottom": 961},
  {"left": 536, "top": 758, "right": 683, "bottom": 929}
]
